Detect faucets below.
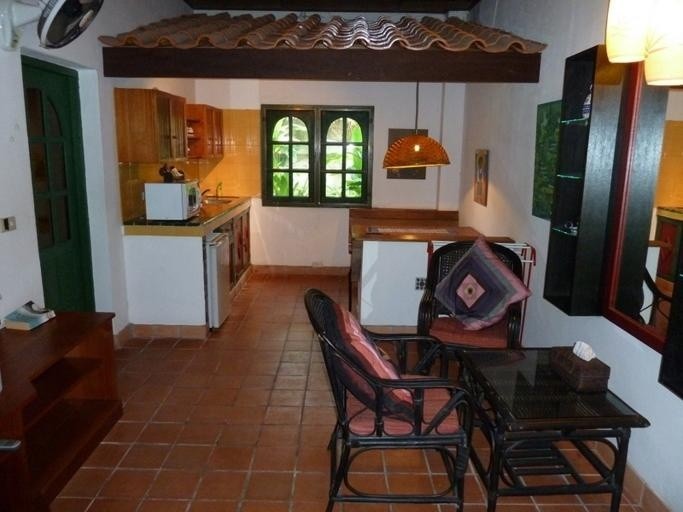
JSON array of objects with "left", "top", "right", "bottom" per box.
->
[{"left": 201, "top": 189, "right": 211, "bottom": 196}]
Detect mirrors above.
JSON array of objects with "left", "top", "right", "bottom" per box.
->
[{"left": 600, "top": 57, "right": 683, "bottom": 356}]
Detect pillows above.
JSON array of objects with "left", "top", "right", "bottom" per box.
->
[
  {"left": 435, "top": 236, "right": 533, "bottom": 332},
  {"left": 322, "top": 293, "right": 419, "bottom": 426}
]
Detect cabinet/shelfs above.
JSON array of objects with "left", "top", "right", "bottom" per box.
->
[
  {"left": 186, "top": 103, "right": 222, "bottom": 160},
  {"left": 0, "top": 309, "right": 126, "bottom": 512},
  {"left": 114, "top": 87, "right": 188, "bottom": 165},
  {"left": 544, "top": 44, "right": 634, "bottom": 320},
  {"left": 221, "top": 207, "right": 251, "bottom": 290}
]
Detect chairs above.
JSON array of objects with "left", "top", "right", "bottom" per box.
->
[
  {"left": 302, "top": 287, "right": 475, "bottom": 512},
  {"left": 415, "top": 240, "right": 525, "bottom": 380}
]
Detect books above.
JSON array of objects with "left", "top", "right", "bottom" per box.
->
[{"left": 3, "top": 301, "right": 56, "bottom": 331}]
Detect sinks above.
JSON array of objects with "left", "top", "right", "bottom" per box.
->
[{"left": 204, "top": 199, "right": 232, "bottom": 205}]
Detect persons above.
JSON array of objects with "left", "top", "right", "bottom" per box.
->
[{"left": 474, "top": 152, "right": 485, "bottom": 204}]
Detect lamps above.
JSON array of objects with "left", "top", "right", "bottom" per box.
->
[
  {"left": 606, "top": 0, "right": 683, "bottom": 88},
  {"left": 381, "top": 80, "right": 451, "bottom": 169}
]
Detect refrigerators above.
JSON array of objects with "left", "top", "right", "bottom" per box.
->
[{"left": 207, "top": 233, "right": 231, "bottom": 329}]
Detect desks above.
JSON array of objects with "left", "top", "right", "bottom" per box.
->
[
  {"left": 455, "top": 346, "right": 651, "bottom": 512},
  {"left": 348, "top": 222, "right": 485, "bottom": 315}
]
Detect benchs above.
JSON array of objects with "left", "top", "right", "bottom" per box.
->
[{"left": 349, "top": 207, "right": 458, "bottom": 287}]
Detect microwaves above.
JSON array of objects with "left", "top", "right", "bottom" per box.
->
[{"left": 144, "top": 180, "right": 202, "bottom": 220}]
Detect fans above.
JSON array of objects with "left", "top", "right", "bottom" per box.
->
[{"left": 0, "top": 0, "right": 108, "bottom": 54}]
